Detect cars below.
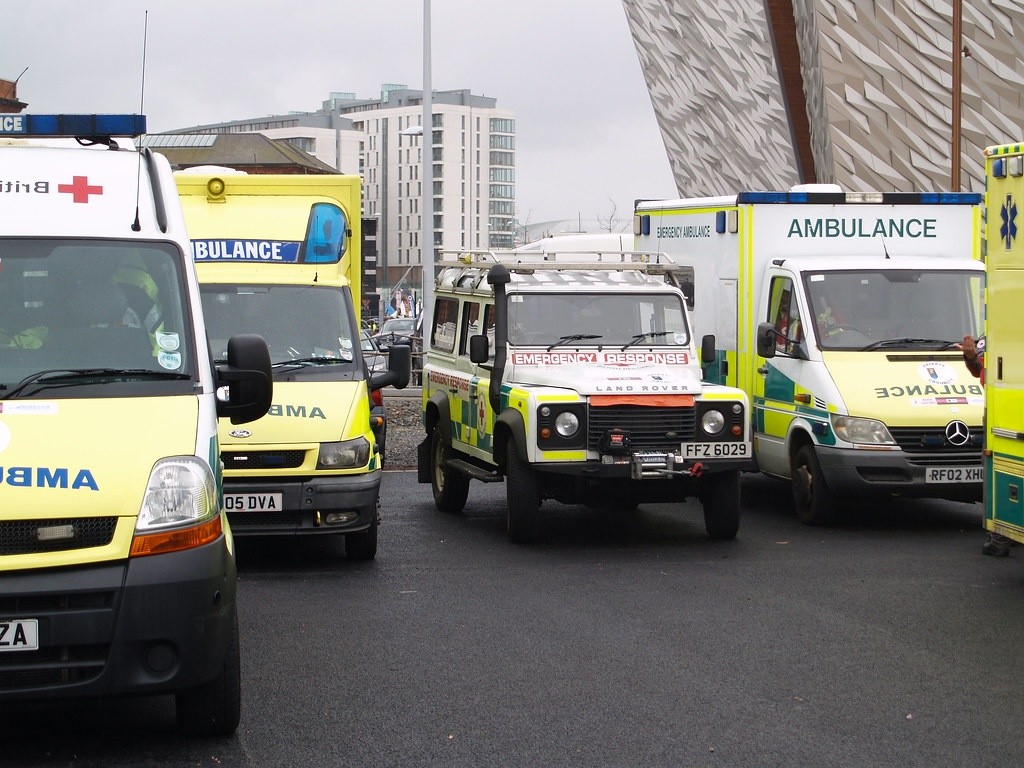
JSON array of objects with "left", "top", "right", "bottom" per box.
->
[
  {"left": 360, "top": 331, "right": 386, "bottom": 379},
  {"left": 374, "top": 316, "right": 417, "bottom": 350},
  {"left": 369, "top": 389, "right": 387, "bottom": 470}
]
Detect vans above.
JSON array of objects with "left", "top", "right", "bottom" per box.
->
[
  {"left": 411, "top": 306, "right": 423, "bottom": 386},
  {"left": 491, "top": 234, "right": 634, "bottom": 260}
]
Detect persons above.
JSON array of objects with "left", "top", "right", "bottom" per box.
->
[
  {"left": 384, "top": 294, "right": 412, "bottom": 318},
  {"left": 954, "top": 335, "right": 1016, "bottom": 555},
  {"left": 6, "top": 253, "right": 166, "bottom": 358},
  {"left": 395, "top": 288, "right": 422, "bottom": 314},
  {"left": 777, "top": 281, "right": 850, "bottom": 349}
]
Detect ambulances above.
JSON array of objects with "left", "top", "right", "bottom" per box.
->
[
  {"left": 415, "top": 247, "right": 752, "bottom": 543},
  {"left": 982, "top": 142, "right": 1024, "bottom": 543},
  {"left": 169, "top": 167, "right": 410, "bottom": 561},
  {"left": 636, "top": 182, "right": 987, "bottom": 527},
  {"left": 0, "top": 114, "right": 273, "bottom": 746}
]
